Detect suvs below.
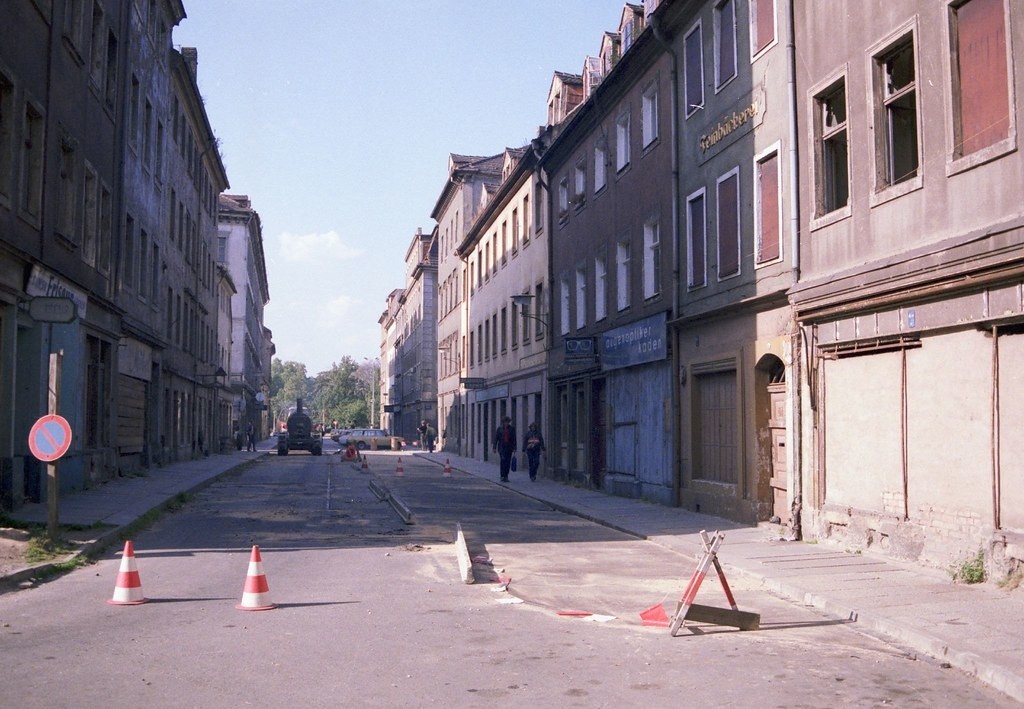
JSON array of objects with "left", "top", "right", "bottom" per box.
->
[{"left": 347, "top": 429, "right": 403, "bottom": 450}]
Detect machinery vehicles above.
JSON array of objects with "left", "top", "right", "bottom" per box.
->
[{"left": 277, "top": 398, "right": 324, "bottom": 456}]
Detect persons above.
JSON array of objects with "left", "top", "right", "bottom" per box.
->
[
  {"left": 492, "top": 416, "right": 517, "bottom": 483},
  {"left": 246, "top": 419, "right": 258, "bottom": 452},
  {"left": 417, "top": 420, "right": 426, "bottom": 451},
  {"left": 425, "top": 423, "right": 436, "bottom": 454},
  {"left": 522, "top": 422, "right": 546, "bottom": 482}
]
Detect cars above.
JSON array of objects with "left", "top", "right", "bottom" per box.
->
[{"left": 331, "top": 429, "right": 351, "bottom": 445}]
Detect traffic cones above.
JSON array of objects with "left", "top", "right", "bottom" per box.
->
[
  {"left": 442, "top": 458, "right": 452, "bottom": 478},
  {"left": 107, "top": 540, "right": 150, "bottom": 605},
  {"left": 395, "top": 457, "right": 406, "bottom": 476},
  {"left": 235, "top": 544, "right": 278, "bottom": 611},
  {"left": 361, "top": 454, "right": 368, "bottom": 468}
]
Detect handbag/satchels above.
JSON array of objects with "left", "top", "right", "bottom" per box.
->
[
  {"left": 249, "top": 431, "right": 253, "bottom": 438},
  {"left": 511, "top": 456, "right": 517, "bottom": 472}
]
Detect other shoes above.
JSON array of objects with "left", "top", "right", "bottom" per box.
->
[
  {"left": 531, "top": 477, "right": 536, "bottom": 481},
  {"left": 504, "top": 480, "right": 510, "bottom": 482}
]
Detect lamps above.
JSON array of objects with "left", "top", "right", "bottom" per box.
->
[
  {"left": 509, "top": 294, "right": 549, "bottom": 336},
  {"left": 438, "top": 346, "right": 461, "bottom": 373}
]
